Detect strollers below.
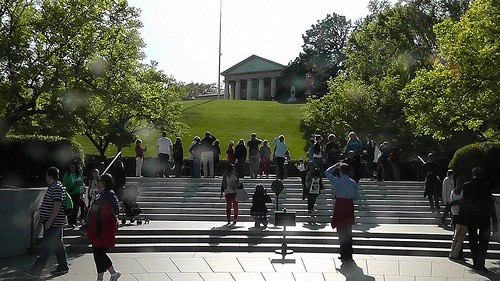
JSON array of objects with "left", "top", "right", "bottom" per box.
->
[{"left": 121, "top": 185, "right": 149, "bottom": 225}]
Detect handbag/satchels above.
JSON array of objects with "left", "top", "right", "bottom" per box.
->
[
  {"left": 309, "top": 178, "right": 319, "bottom": 194},
  {"left": 63, "top": 192, "right": 73, "bottom": 216},
  {"left": 373, "top": 146, "right": 383, "bottom": 163},
  {"left": 235, "top": 186, "right": 249, "bottom": 202}
]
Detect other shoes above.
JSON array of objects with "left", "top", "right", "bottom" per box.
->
[
  {"left": 338, "top": 256, "right": 343, "bottom": 259},
  {"left": 24, "top": 269, "right": 41, "bottom": 278},
  {"left": 309, "top": 209, "right": 314, "bottom": 213},
  {"left": 228, "top": 222, "right": 236, "bottom": 227},
  {"left": 110, "top": 272, "right": 121, "bottom": 281},
  {"left": 363, "top": 177, "right": 374, "bottom": 181},
  {"left": 450, "top": 256, "right": 464, "bottom": 261},
  {"left": 476, "top": 266, "right": 489, "bottom": 273},
  {"left": 50, "top": 268, "right": 68, "bottom": 274},
  {"left": 255, "top": 222, "right": 259, "bottom": 227}
]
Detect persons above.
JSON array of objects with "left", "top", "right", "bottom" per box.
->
[
  {"left": 23, "top": 166, "right": 68, "bottom": 276},
  {"left": 461, "top": 168, "right": 497, "bottom": 269},
  {"left": 85, "top": 174, "right": 121, "bottom": 280},
  {"left": 133, "top": 139, "right": 148, "bottom": 177},
  {"left": 301, "top": 161, "right": 323, "bottom": 215},
  {"left": 186, "top": 131, "right": 402, "bottom": 182},
  {"left": 156, "top": 130, "right": 173, "bottom": 178},
  {"left": 324, "top": 161, "right": 359, "bottom": 263},
  {"left": 439, "top": 166, "right": 454, "bottom": 227},
  {"left": 62, "top": 155, "right": 128, "bottom": 228},
  {"left": 220, "top": 163, "right": 242, "bottom": 228},
  {"left": 418, "top": 152, "right": 441, "bottom": 215},
  {"left": 248, "top": 182, "right": 273, "bottom": 229},
  {"left": 172, "top": 136, "right": 184, "bottom": 177},
  {"left": 446, "top": 174, "right": 471, "bottom": 261}
]
